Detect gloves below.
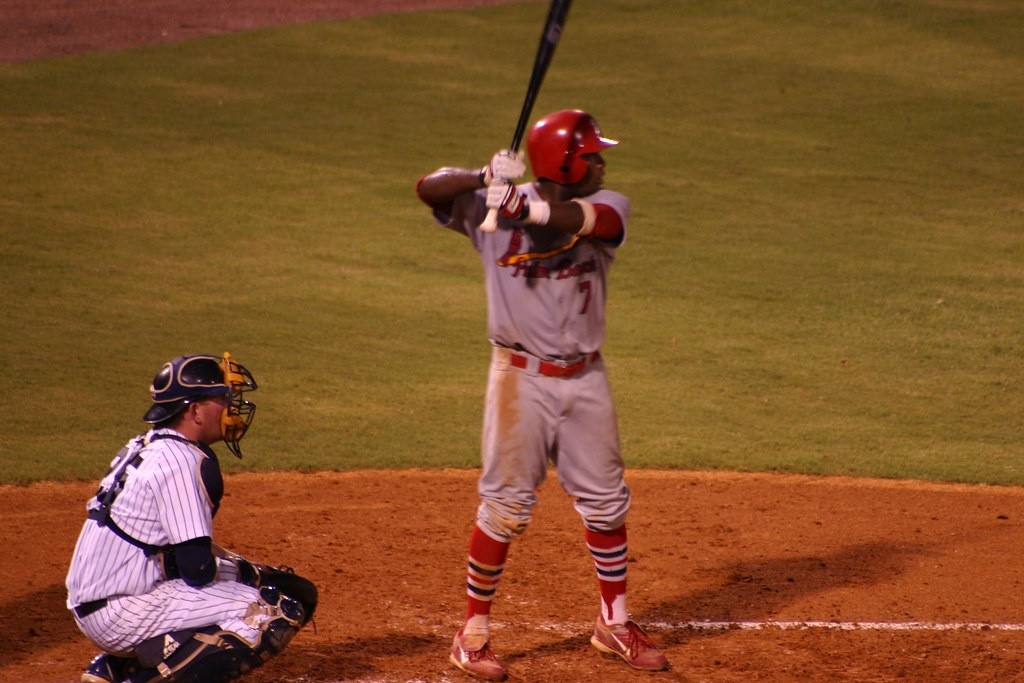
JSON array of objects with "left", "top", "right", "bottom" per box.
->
[
  {"left": 485, "top": 179, "right": 550, "bottom": 226},
  {"left": 479, "top": 149, "right": 526, "bottom": 188}
]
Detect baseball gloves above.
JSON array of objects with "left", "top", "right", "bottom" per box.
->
[{"left": 227, "top": 549, "right": 319, "bottom": 627}]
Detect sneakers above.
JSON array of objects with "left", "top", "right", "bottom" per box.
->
[
  {"left": 449, "top": 626, "right": 505, "bottom": 681},
  {"left": 590, "top": 614, "right": 665, "bottom": 670}
]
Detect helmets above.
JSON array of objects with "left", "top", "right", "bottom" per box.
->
[
  {"left": 525, "top": 109, "right": 619, "bottom": 184},
  {"left": 142, "top": 353, "right": 243, "bottom": 424}
]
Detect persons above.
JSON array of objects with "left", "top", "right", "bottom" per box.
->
[
  {"left": 65, "top": 352, "right": 319, "bottom": 683},
  {"left": 415, "top": 108, "right": 666, "bottom": 681}
]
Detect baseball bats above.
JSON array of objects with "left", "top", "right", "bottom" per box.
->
[{"left": 480, "top": 0, "right": 574, "bottom": 233}]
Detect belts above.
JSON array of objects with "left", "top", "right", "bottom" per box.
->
[
  {"left": 74, "top": 598, "right": 107, "bottom": 618},
  {"left": 511, "top": 350, "right": 600, "bottom": 379}
]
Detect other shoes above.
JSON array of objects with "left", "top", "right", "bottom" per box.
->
[{"left": 81, "top": 651, "right": 141, "bottom": 683}]
